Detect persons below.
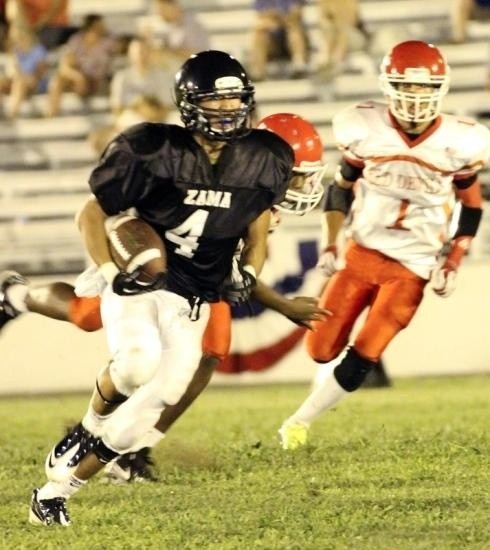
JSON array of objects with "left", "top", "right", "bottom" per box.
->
[
  {"left": 0, "top": 108, "right": 336, "bottom": 486},
  {"left": 27, "top": 46, "right": 298, "bottom": 534},
  {"left": 1, "top": 1, "right": 220, "bottom": 166},
  {"left": 275, "top": 37, "right": 490, "bottom": 454},
  {"left": 245, "top": 0, "right": 375, "bottom": 82},
  {"left": 448, "top": 1, "right": 490, "bottom": 43}
]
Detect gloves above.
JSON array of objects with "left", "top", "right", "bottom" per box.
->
[
  {"left": 430, "top": 263, "right": 458, "bottom": 299},
  {"left": 112, "top": 265, "right": 167, "bottom": 296},
  {"left": 222, "top": 265, "right": 258, "bottom": 308},
  {"left": 318, "top": 245, "right": 338, "bottom": 278}
]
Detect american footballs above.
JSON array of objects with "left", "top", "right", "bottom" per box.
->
[{"left": 103, "top": 214, "right": 167, "bottom": 282}]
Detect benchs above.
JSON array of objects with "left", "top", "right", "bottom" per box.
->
[{"left": 0, "top": 0, "right": 490, "bottom": 273}]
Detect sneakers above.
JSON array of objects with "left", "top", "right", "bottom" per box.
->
[
  {"left": 0, "top": 270, "right": 29, "bottom": 336},
  {"left": 28, "top": 488, "right": 71, "bottom": 529},
  {"left": 276, "top": 419, "right": 311, "bottom": 450},
  {"left": 42, "top": 422, "right": 98, "bottom": 485},
  {"left": 108, "top": 445, "right": 156, "bottom": 487}
]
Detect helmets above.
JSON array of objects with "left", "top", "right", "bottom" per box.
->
[
  {"left": 256, "top": 112, "right": 324, "bottom": 214},
  {"left": 173, "top": 49, "right": 256, "bottom": 142},
  {"left": 379, "top": 40, "right": 453, "bottom": 125}
]
